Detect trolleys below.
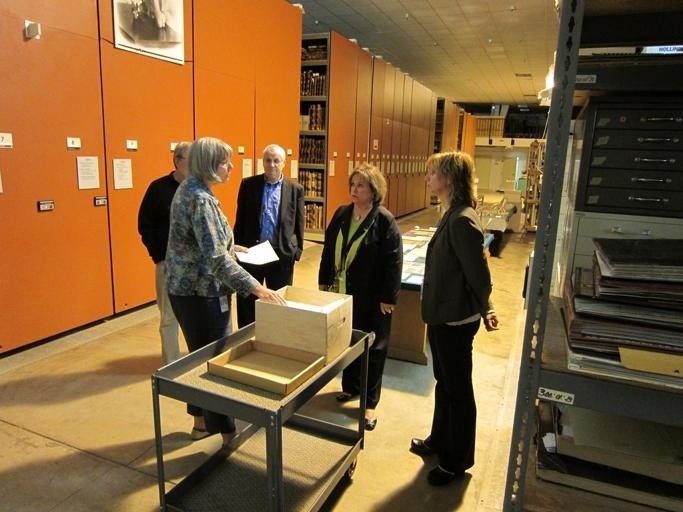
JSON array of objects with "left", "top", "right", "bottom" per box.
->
[{"left": 151, "top": 322, "right": 376, "bottom": 512}]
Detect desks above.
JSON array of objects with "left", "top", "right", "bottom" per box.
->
[
  {"left": 476, "top": 201, "right": 517, "bottom": 257},
  {"left": 385, "top": 228, "right": 495, "bottom": 365}
]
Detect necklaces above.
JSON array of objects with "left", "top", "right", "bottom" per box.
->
[{"left": 354, "top": 208, "right": 368, "bottom": 221}]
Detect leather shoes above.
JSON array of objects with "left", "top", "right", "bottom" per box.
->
[
  {"left": 365, "top": 417, "right": 376, "bottom": 430},
  {"left": 412, "top": 439, "right": 432, "bottom": 454},
  {"left": 337, "top": 393, "right": 355, "bottom": 402},
  {"left": 191, "top": 428, "right": 210, "bottom": 439},
  {"left": 427, "top": 466, "right": 455, "bottom": 486}
]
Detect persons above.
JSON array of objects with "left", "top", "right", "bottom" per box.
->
[
  {"left": 232, "top": 144, "right": 305, "bottom": 331},
  {"left": 411, "top": 151, "right": 499, "bottom": 487},
  {"left": 318, "top": 165, "right": 403, "bottom": 430},
  {"left": 164, "top": 137, "right": 288, "bottom": 442},
  {"left": 138, "top": 142, "right": 191, "bottom": 363}
]
